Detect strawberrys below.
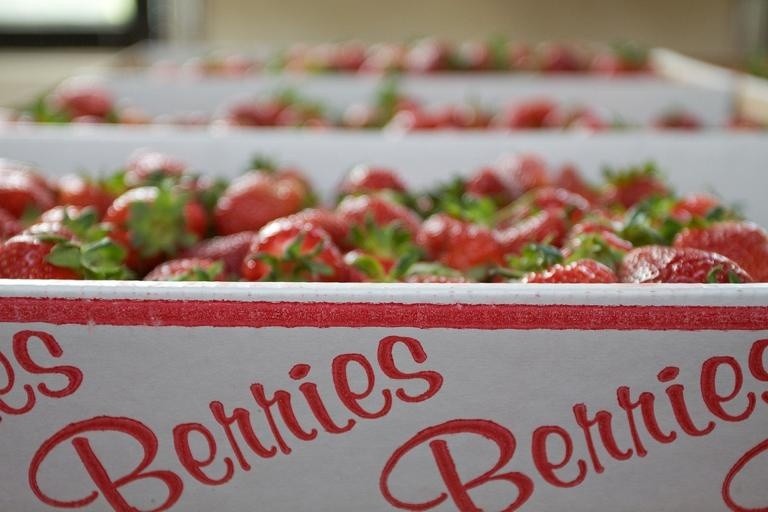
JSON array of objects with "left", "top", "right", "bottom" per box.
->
[{"left": 0, "top": 33, "right": 768, "bottom": 283}]
